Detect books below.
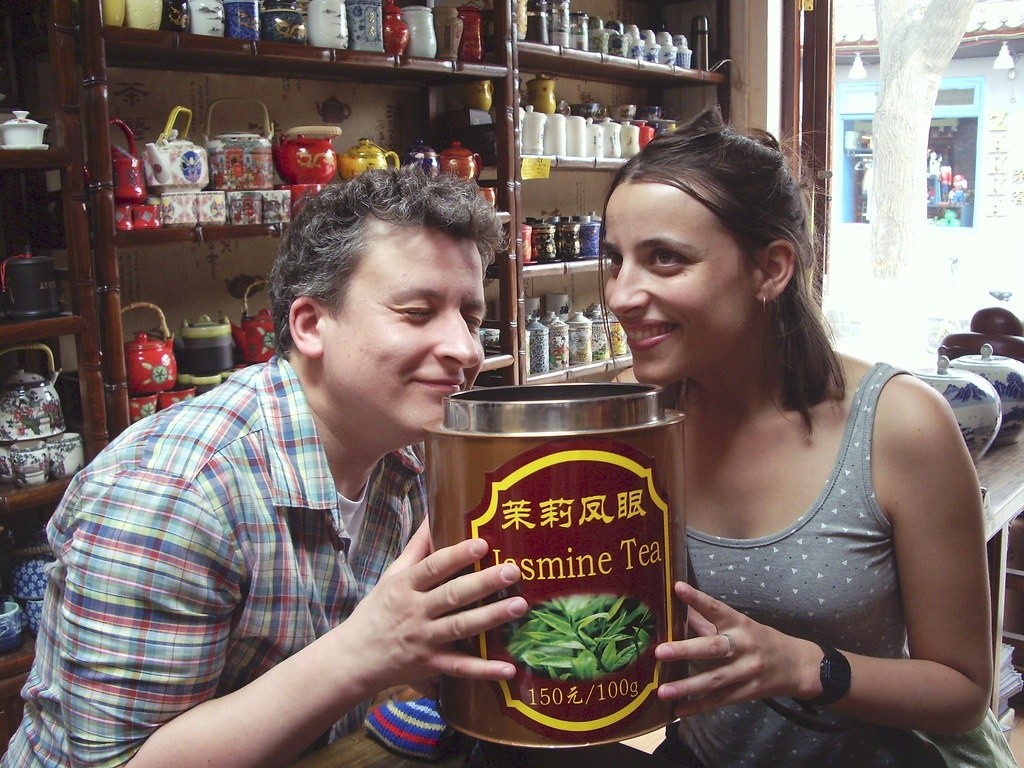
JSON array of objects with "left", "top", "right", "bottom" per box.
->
[{"left": 998, "top": 643, "right": 1023, "bottom": 732}]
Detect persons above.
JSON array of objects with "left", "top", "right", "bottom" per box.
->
[
  {"left": 1, "top": 163, "right": 528, "bottom": 768},
  {"left": 599, "top": 107, "right": 994, "bottom": 768},
  {"left": 939, "top": 160, "right": 965, "bottom": 206}
]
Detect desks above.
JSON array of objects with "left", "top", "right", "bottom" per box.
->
[{"left": 976, "top": 428, "right": 1024, "bottom": 717}]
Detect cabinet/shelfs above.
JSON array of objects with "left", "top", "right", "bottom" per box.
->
[
  {"left": 848, "top": 147, "right": 974, "bottom": 227},
  {"left": 0, "top": 0, "right": 744, "bottom": 758}
]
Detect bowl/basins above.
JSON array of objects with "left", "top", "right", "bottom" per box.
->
[
  {"left": 637, "top": 105, "right": 662, "bottom": 121},
  {"left": 608, "top": 104, "right": 636, "bottom": 122},
  {"left": 569, "top": 102, "right": 601, "bottom": 119},
  {"left": 519, "top": 73, "right": 655, "bottom": 159}
]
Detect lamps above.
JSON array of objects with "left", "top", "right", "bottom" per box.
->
[
  {"left": 992, "top": 42, "right": 1019, "bottom": 72},
  {"left": 848, "top": 52, "right": 867, "bottom": 79}
]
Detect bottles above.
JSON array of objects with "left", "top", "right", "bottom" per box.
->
[
  {"left": 525, "top": 0, "right": 590, "bottom": 52},
  {"left": 420, "top": 382, "right": 689, "bottom": 751},
  {"left": 523, "top": 304, "right": 629, "bottom": 374},
  {"left": 950, "top": 343, "right": 1024, "bottom": 448},
  {"left": 916, "top": 357, "right": 1001, "bottom": 465},
  {"left": 521, "top": 211, "right": 604, "bottom": 264}
]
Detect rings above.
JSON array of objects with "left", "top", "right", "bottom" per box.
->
[{"left": 722, "top": 633, "right": 736, "bottom": 659}]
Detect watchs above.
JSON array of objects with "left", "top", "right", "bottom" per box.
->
[{"left": 791, "top": 638, "right": 851, "bottom": 707}]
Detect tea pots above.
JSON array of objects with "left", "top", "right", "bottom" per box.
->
[
  {"left": 9, "top": 544, "right": 56, "bottom": 601},
  {"left": 121, "top": 279, "right": 277, "bottom": 396},
  {"left": 110, "top": 98, "right": 483, "bottom": 206},
  {"left": 0, "top": 243, "right": 61, "bottom": 322},
  {"left": 0, "top": 341, "right": 67, "bottom": 441}
]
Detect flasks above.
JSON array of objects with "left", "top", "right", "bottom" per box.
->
[{"left": 689, "top": 17, "right": 709, "bottom": 71}]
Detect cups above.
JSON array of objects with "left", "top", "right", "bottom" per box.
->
[
  {"left": 0, "top": 602, "right": 24, "bottom": 654},
  {"left": 100, "top": 0, "right": 486, "bottom": 66},
  {"left": 546, "top": 292, "right": 572, "bottom": 322},
  {"left": 588, "top": 15, "right": 692, "bottom": 71},
  {"left": 129, "top": 368, "right": 238, "bottom": 425},
  {"left": 24, "top": 601, "right": 44, "bottom": 637},
  {"left": 116, "top": 183, "right": 323, "bottom": 232},
  {"left": 0, "top": 433, "right": 85, "bottom": 488},
  {"left": 525, "top": 297, "right": 541, "bottom": 320},
  {"left": 0, "top": 109, "right": 50, "bottom": 151}
]
